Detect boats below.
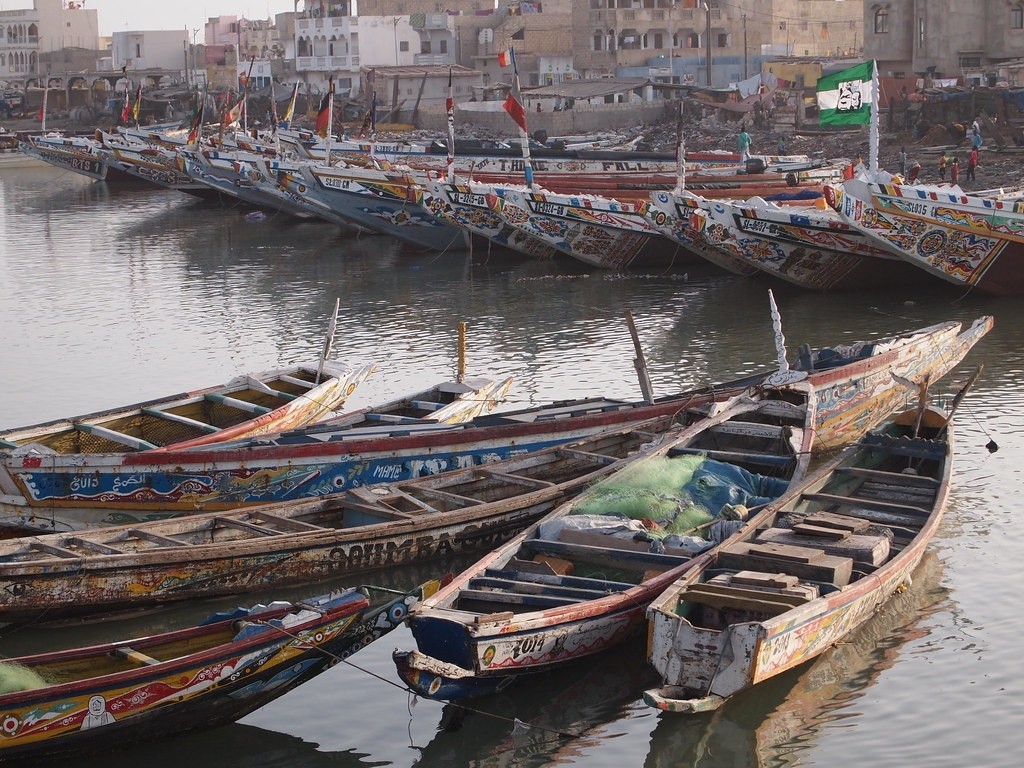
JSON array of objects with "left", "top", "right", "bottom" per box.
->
[{"left": 2, "top": 113, "right": 1024, "bottom": 768}]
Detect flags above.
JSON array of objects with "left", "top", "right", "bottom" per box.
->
[
  {"left": 121, "top": 83, "right": 132, "bottom": 123},
  {"left": 277, "top": 80, "right": 297, "bottom": 130},
  {"left": 132, "top": 80, "right": 141, "bottom": 121},
  {"left": 38, "top": 93, "right": 44, "bottom": 122},
  {"left": 502, "top": 65, "right": 527, "bottom": 134},
  {"left": 497, "top": 45, "right": 514, "bottom": 67},
  {"left": 815, "top": 59, "right": 872, "bottom": 126},
  {"left": 270, "top": 86, "right": 278, "bottom": 136},
  {"left": 186, "top": 69, "right": 247, "bottom": 144},
  {"left": 315, "top": 90, "right": 328, "bottom": 139}
]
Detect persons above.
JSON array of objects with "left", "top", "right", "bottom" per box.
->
[
  {"left": 166, "top": 99, "right": 185, "bottom": 118},
  {"left": 536, "top": 102, "right": 571, "bottom": 113},
  {"left": 898, "top": 119, "right": 983, "bottom": 185},
  {"left": 737, "top": 128, "right": 753, "bottom": 166},
  {"left": 264, "top": 110, "right": 270, "bottom": 125},
  {"left": 6, "top": 99, "right": 15, "bottom": 112},
  {"left": 777, "top": 136, "right": 789, "bottom": 156}
]
